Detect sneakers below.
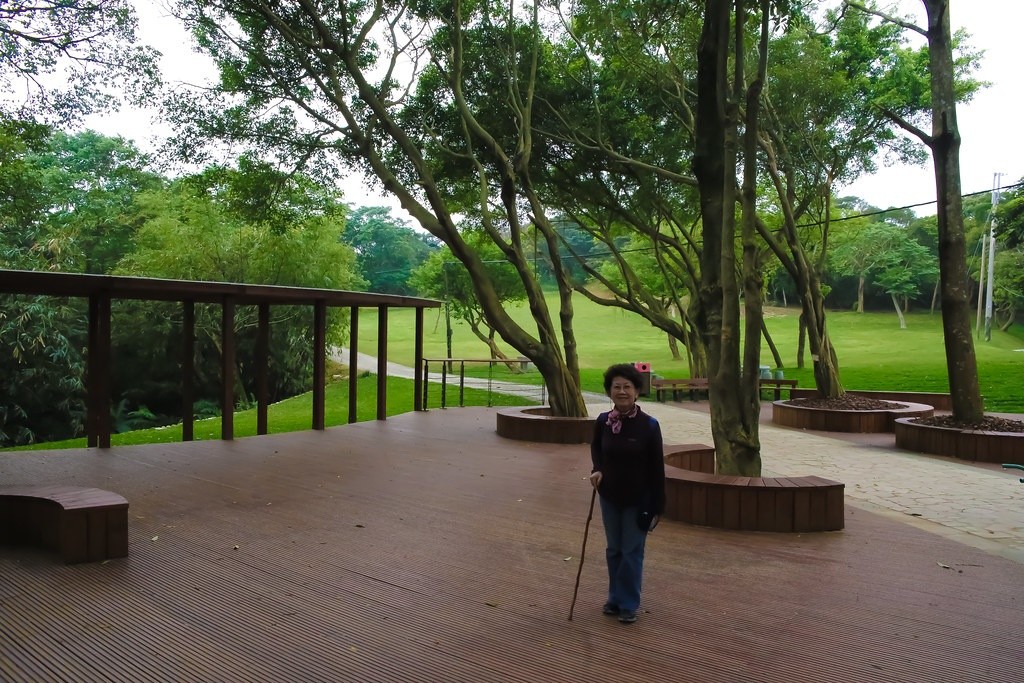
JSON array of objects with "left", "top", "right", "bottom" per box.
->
[
  {"left": 617, "top": 610, "right": 636, "bottom": 622},
  {"left": 603, "top": 602, "right": 618, "bottom": 614}
]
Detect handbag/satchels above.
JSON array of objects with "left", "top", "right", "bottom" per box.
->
[{"left": 638, "top": 510, "right": 661, "bottom": 532}]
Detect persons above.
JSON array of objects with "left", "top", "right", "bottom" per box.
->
[{"left": 590, "top": 363, "right": 664, "bottom": 621}]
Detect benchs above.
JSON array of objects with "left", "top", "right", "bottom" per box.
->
[{"left": 651, "top": 378, "right": 798, "bottom": 403}]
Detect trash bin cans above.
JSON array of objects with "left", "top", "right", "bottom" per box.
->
[{"left": 635, "top": 363, "right": 650, "bottom": 396}]
